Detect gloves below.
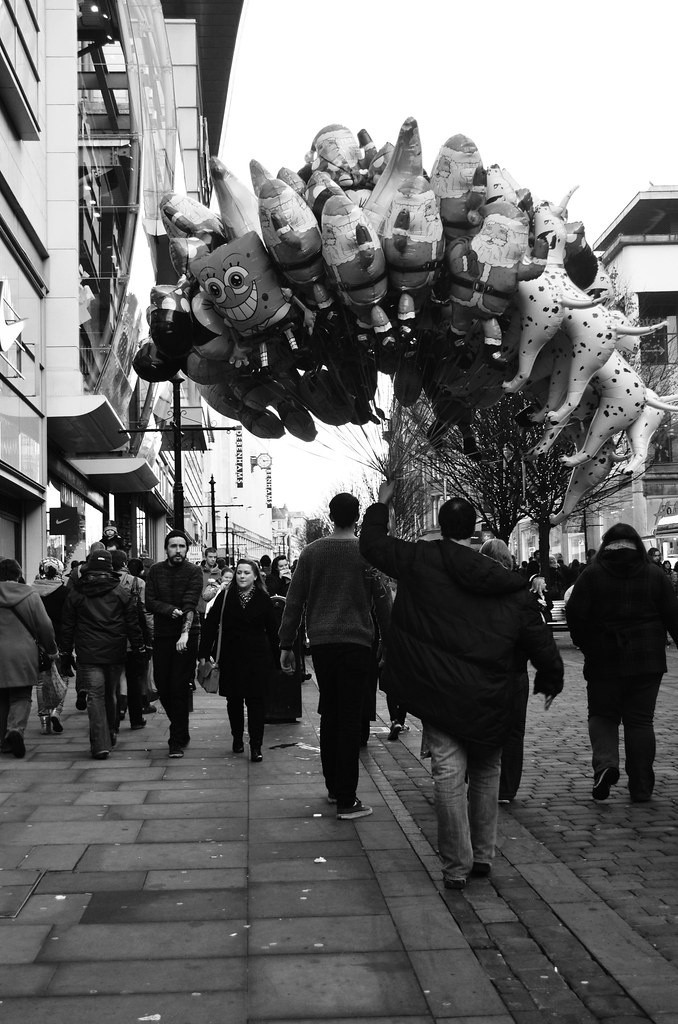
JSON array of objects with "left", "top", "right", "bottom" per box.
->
[
  {"left": 135, "top": 646, "right": 148, "bottom": 666},
  {"left": 61, "top": 654, "right": 78, "bottom": 676}
]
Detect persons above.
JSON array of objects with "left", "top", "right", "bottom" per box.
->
[
  {"left": 648, "top": 547, "right": 678, "bottom": 645},
  {"left": 279, "top": 493, "right": 409, "bottom": 819},
  {"left": 195, "top": 547, "right": 312, "bottom": 761},
  {"left": 358, "top": 480, "right": 564, "bottom": 888},
  {"left": 145, "top": 532, "right": 204, "bottom": 757},
  {"left": 567, "top": 524, "right": 678, "bottom": 802},
  {"left": 514, "top": 547, "right": 596, "bottom": 622},
  {"left": 480, "top": 539, "right": 529, "bottom": 804},
  {"left": 0, "top": 526, "right": 154, "bottom": 758}
]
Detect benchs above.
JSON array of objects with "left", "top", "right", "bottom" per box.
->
[{"left": 546, "top": 600, "right": 567, "bottom": 631}]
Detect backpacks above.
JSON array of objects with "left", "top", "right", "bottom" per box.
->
[{"left": 129, "top": 576, "right": 146, "bottom": 632}]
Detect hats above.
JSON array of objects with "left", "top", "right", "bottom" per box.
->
[
  {"left": 103, "top": 520, "right": 118, "bottom": 535},
  {"left": 87, "top": 550, "right": 113, "bottom": 570}
]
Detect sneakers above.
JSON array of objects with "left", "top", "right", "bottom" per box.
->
[{"left": 338, "top": 796, "right": 372, "bottom": 821}]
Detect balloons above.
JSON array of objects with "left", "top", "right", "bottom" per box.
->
[{"left": 134, "top": 117, "right": 678, "bottom": 523}]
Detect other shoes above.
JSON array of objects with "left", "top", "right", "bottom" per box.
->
[
  {"left": 387, "top": 723, "right": 402, "bottom": 741},
  {"left": 8, "top": 730, "right": 28, "bottom": 757},
  {"left": 232, "top": 738, "right": 244, "bottom": 752},
  {"left": 443, "top": 878, "right": 463, "bottom": 888},
  {"left": 498, "top": 795, "right": 513, "bottom": 803},
  {"left": 94, "top": 750, "right": 110, "bottom": 760},
  {"left": 167, "top": 745, "right": 185, "bottom": 758},
  {"left": 474, "top": 862, "right": 490, "bottom": 875},
  {"left": 76, "top": 690, "right": 87, "bottom": 712},
  {"left": 592, "top": 768, "right": 619, "bottom": 801},
  {"left": 251, "top": 747, "right": 263, "bottom": 762}
]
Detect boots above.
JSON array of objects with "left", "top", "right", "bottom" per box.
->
[
  {"left": 49, "top": 708, "right": 64, "bottom": 732},
  {"left": 40, "top": 715, "right": 49, "bottom": 734},
  {"left": 142, "top": 693, "right": 157, "bottom": 715},
  {"left": 120, "top": 694, "right": 128, "bottom": 720}
]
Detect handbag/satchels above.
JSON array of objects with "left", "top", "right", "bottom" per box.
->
[
  {"left": 196, "top": 660, "right": 220, "bottom": 694},
  {"left": 35, "top": 643, "right": 50, "bottom": 672},
  {"left": 42, "top": 661, "right": 66, "bottom": 711}
]
